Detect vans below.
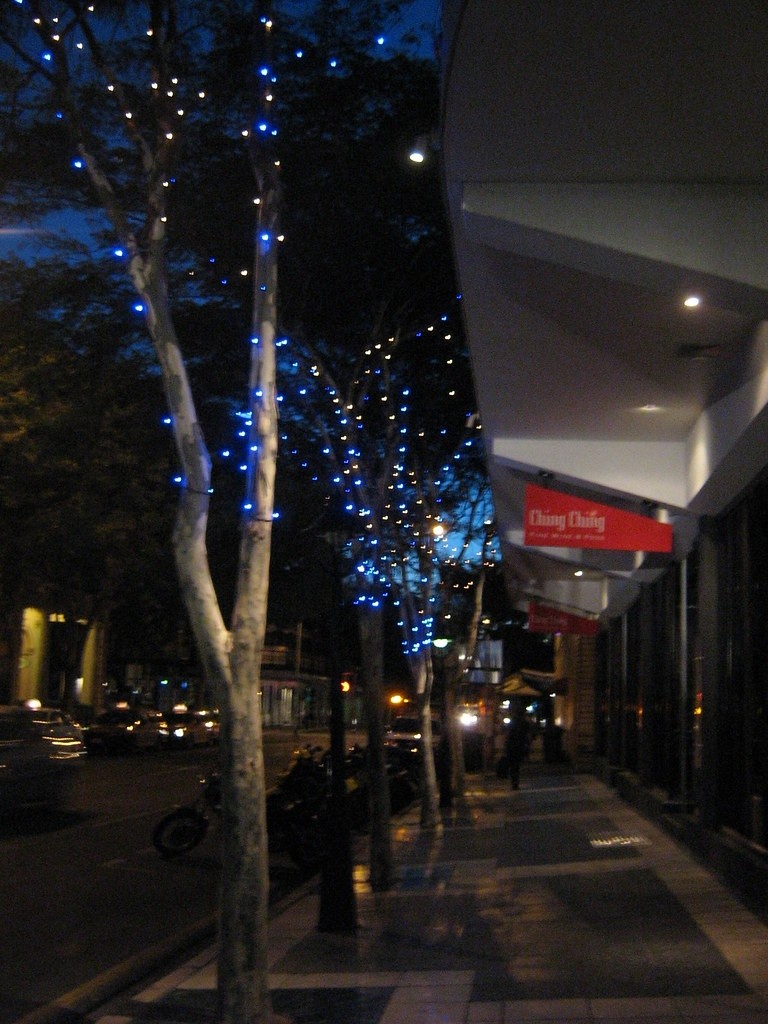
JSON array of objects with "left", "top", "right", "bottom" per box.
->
[{"left": 384, "top": 718, "right": 440, "bottom": 751}]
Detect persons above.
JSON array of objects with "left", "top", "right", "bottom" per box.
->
[{"left": 504, "top": 705, "right": 530, "bottom": 790}]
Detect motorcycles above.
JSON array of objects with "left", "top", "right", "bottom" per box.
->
[{"left": 151, "top": 744, "right": 417, "bottom": 855}]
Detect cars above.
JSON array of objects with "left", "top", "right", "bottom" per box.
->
[
  {"left": 85, "top": 710, "right": 218, "bottom": 750},
  {"left": 0, "top": 707, "right": 87, "bottom": 782}
]
